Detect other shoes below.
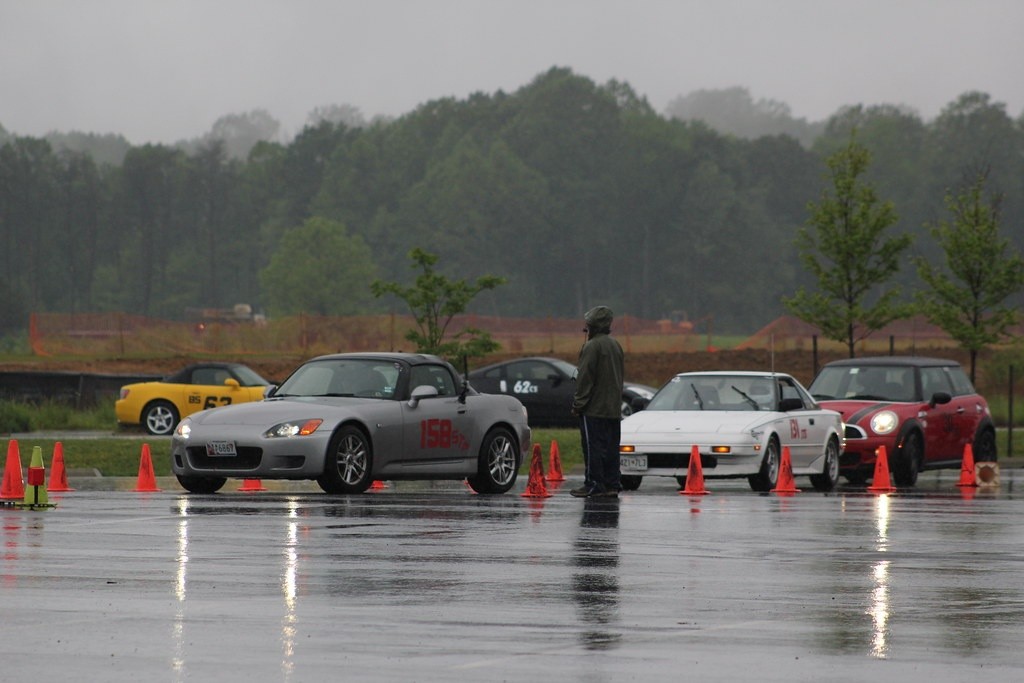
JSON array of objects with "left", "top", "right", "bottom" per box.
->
[
  {"left": 602, "top": 485, "right": 619, "bottom": 496},
  {"left": 570, "top": 486, "right": 603, "bottom": 497}
]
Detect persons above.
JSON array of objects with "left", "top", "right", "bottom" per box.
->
[{"left": 570, "top": 307, "right": 624, "bottom": 497}]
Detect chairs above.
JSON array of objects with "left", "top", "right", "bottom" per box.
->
[
  {"left": 749, "top": 379, "right": 769, "bottom": 396},
  {"left": 685, "top": 384, "right": 719, "bottom": 409}
]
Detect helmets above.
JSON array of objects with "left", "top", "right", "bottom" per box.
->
[
  {"left": 902, "top": 371, "right": 927, "bottom": 392},
  {"left": 748, "top": 380, "right": 773, "bottom": 405},
  {"left": 856, "top": 366, "right": 886, "bottom": 386}
]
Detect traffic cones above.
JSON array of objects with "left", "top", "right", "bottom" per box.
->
[
  {"left": 238, "top": 479, "right": 267, "bottom": 491},
  {"left": 867, "top": 445, "right": 895, "bottom": 489},
  {"left": 546, "top": 440, "right": 566, "bottom": 481},
  {"left": 679, "top": 445, "right": 711, "bottom": 495},
  {"left": 130, "top": 443, "right": 163, "bottom": 492},
  {"left": 526, "top": 498, "right": 547, "bottom": 528},
  {"left": 368, "top": 480, "right": 389, "bottom": 490},
  {"left": 45, "top": 442, "right": 76, "bottom": 492},
  {"left": 956, "top": 442, "right": 979, "bottom": 485},
  {"left": 960, "top": 486, "right": 976, "bottom": 500},
  {"left": 520, "top": 443, "right": 554, "bottom": 497},
  {"left": 16, "top": 446, "right": 53, "bottom": 510},
  {"left": 0, "top": 440, "right": 26, "bottom": 502},
  {"left": 769, "top": 445, "right": 802, "bottom": 496}
]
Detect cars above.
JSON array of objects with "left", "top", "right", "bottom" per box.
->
[
  {"left": 808, "top": 356, "right": 997, "bottom": 486},
  {"left": 620, "top": 371, "right": 846, "bottom": 491},
  {"left": 459, "top": 357, "right": 659, "bottom": 426},
  {"left": 116, "top": 363, "right": 278, "bottom": 436},
  {"left": 171, "top": 348, "right": 532, "bottom": 494}
]
